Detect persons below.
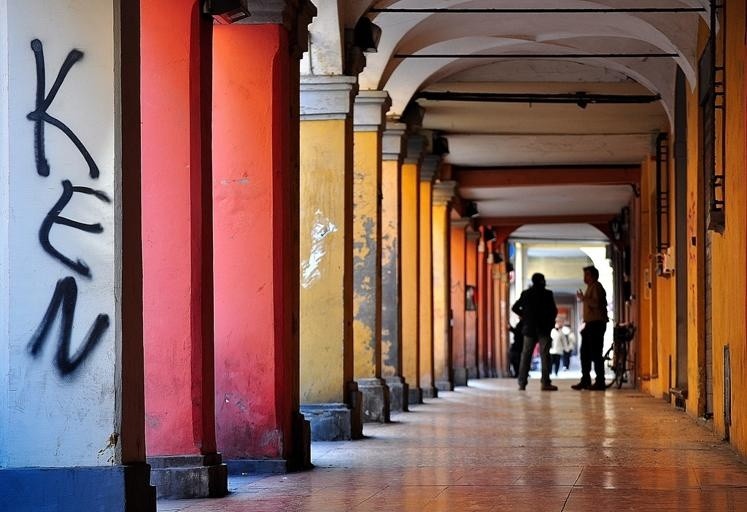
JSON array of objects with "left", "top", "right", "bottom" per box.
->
[
  {"left": 561, "top": 323, "right": 575, "bottom": 371},
  {"left": 548, "top": 320, "right": 570, "bottom": 375},
  {"left": 512, "top": 273, "right": 559, "bottom": 390},
  {"left": 507, "top": 316, "right": 529, "bottom": 378},
  {"left": 570, "top": 265, "right": 609, "bottom": 390}
]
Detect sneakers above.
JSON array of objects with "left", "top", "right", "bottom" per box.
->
[
  {"left": 541, "top": 384, "right": 557, "bottom": 391},
  {"left": 572, "top": 381, "right": 605, "bottom": 390}
]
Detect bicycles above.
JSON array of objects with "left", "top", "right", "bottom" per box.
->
[{"left": 603, "top": 318, "right": 635, "bottom": 391}]
